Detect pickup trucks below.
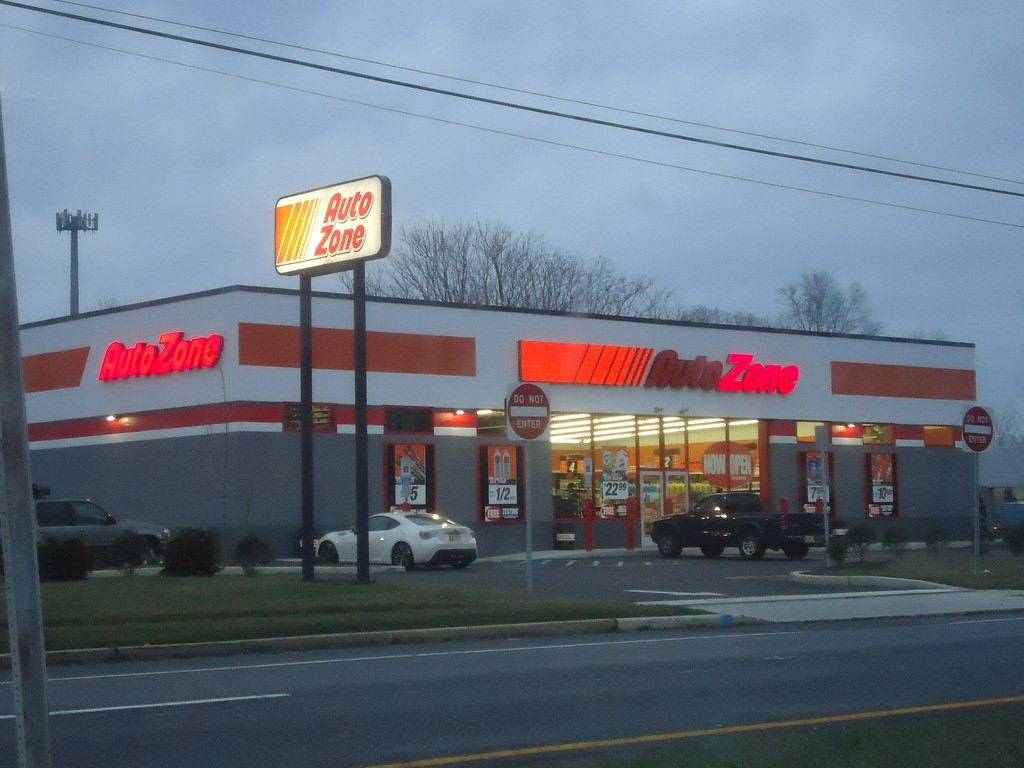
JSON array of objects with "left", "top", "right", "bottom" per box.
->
[{"left": 650, "top": 490, "right": 827, "bottom": 562}]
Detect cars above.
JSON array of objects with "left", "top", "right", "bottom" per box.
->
[
  {"left": 32, "top": 497, "right": 172, "bottom": 570},
  {"left": 315, "top": 511, "right": 478, "bottom": 572}
]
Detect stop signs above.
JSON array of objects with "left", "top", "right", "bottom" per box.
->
[
  {"left": 506, "top": 381, "right": 551, "bottom": 441},
  {"left": 962, "top": 403, "right": 995, "bottom": 454}
]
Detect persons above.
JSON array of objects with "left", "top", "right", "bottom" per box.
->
[
  {"left": 566, "top": 482, "right": 578, "bottom": 516},
  {"left": 612, "top": 503, "right": 620, "bottom": 517}
]
